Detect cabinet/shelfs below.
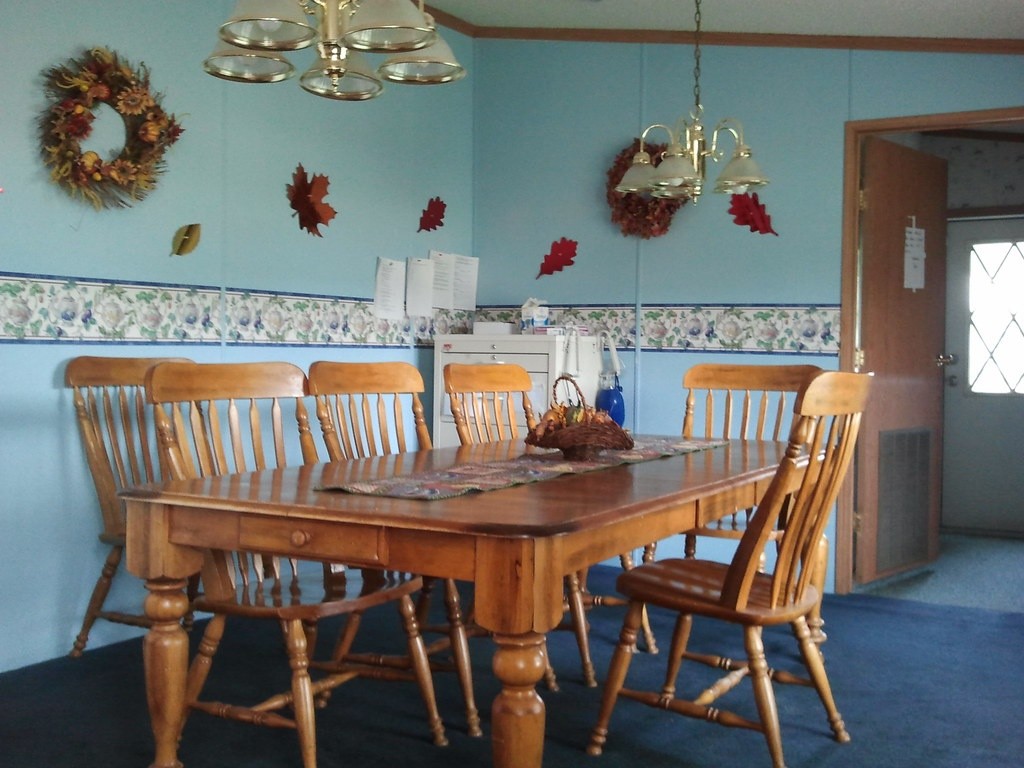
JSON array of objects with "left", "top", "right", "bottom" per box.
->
[{"left": 431, "top": 333, "right": 605, "bottom": 449}]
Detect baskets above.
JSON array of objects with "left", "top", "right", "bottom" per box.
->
[{"left": 526, "top": 376, "right": 635, "bottom": 458}]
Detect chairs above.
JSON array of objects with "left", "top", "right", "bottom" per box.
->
[{"left": 63, "top": 356, "right": 876, "bottom": 768}]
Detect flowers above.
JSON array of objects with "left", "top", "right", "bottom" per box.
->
[{"left": 31, "top": 44, "right": 189, "bottom": 213}]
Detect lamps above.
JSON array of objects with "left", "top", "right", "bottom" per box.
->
[
  {"left": 615, "top": 0, "right": 771, "bottom": 205},
  {"left": 200, "top": 0, "right": 467, "bottom": 102}
]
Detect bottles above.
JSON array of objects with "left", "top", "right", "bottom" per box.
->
[{"left": 597, "top": 371, "right": 625, "bottom": 428}]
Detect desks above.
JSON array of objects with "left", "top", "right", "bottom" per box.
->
[{"left": 113, "top": 433, "right": 835, "bottom": 768}]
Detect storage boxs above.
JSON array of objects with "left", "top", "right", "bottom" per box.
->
[
  {"left": 519, "top": 297, "right": 549, "bottom": 335},
  {"left": 473, "top": 322, "right": 519, "bottom": 335}
]
